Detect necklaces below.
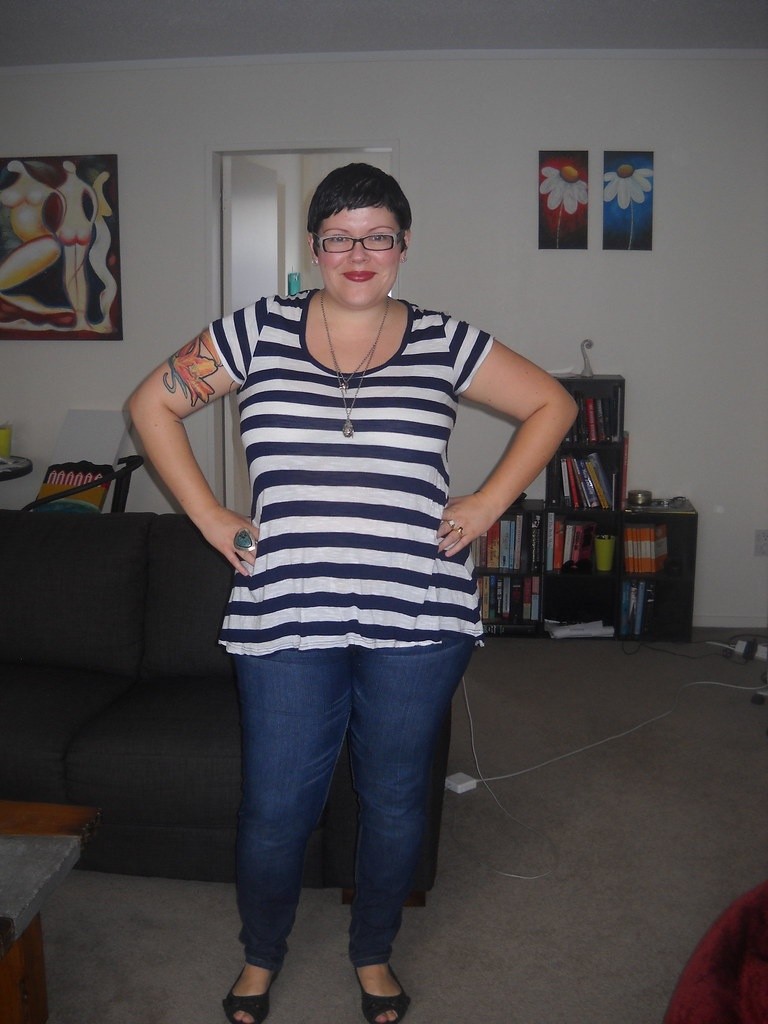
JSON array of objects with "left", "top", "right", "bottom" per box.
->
[{"left": 321, "top": 289, "right": 390, "bottom": 438}]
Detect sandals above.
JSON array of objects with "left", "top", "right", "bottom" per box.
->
[
  {"left": 220, "top": 964, "right": 277, "bottom": 1024},
  {"left": 355, "top": 962, "right": 409, "bottom": 1024}
]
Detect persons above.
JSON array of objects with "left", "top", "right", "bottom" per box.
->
[{"left": 127, "top": 162, "right": 578, "bottom": 1024}]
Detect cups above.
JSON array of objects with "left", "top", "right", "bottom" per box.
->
[
  {"left": 0, "top": 425, "right": 12, "bottom": 458},
  {"left": 594, "top": 535, "right": 615, "bottom": 571}
]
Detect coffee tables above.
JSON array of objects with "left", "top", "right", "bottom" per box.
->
[{"left": 0, "top": 801, "right": 103, "bottom": 1024}]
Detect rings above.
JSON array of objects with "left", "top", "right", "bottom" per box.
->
[
  {"left": 447, "top": 520, "right": 455, "bottom": 528},
  {"left": 234, "top": 527, "right": 256, "bottom": 551},
  {"left": 452, "top": 527, "right": 463, "bottom": 536}
]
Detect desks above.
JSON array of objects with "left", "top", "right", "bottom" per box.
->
[{"left": 0, "top": 455, "right": 32, "bottom": 480}]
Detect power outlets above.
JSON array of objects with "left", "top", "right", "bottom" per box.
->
[{"left": 754, "top": 529, "right": 768, "bottom": 556}]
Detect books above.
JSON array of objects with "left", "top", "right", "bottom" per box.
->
[
  {"left": 469, "top": 513, "right": 668, "bottom": 634},
  {"left": 564, "top": 392, "right": 610, "bottom": 444},
  {"left": 561, "top": 452, "right": 611, "bottom": 509}
]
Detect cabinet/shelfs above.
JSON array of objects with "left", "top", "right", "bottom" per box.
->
[{"left": 472, "top": 375, "right": 699, "bottom": 643}]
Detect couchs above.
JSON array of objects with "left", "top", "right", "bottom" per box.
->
[{"left": 0, "top": 508, "right": 451, "bottom": 909}]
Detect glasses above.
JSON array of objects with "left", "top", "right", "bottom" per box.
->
[{"left": 313, "top": 231, "right": 404, "bottom": 253}]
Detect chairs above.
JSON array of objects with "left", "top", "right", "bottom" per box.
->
[{"left": 18, "top": 455, "right": 144, "bottom": 513}]
